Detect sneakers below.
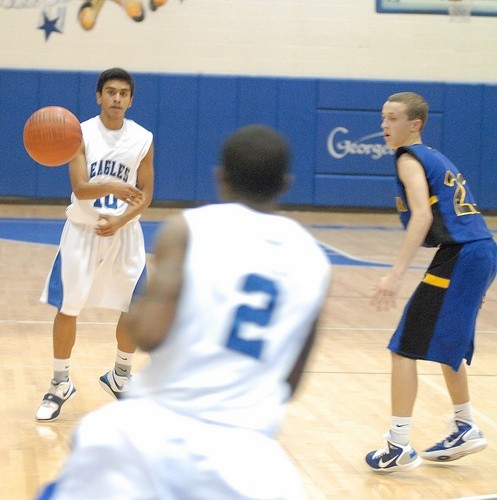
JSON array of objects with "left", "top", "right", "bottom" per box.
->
[
  {"left": 98, "top": 370, "right": 128, "bottom": 401},
  {"left": 36, "top": 378, "right": 77, "bottom": 423},
  {"left": 365, "top": 439, "right": 423, "bottom": 474},
  {"left": 420, "top": 424, "right": 488, "bottom": 463}
]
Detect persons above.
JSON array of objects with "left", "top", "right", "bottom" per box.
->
[
  {"left": 364, "top": 92, "right": 497, "bottom": 471},
  {"left": 35, "top": 68, "right": 154, "bottom": 423},
  {"left": 34, "top": 125, "right": 334, "bottom": 500}
]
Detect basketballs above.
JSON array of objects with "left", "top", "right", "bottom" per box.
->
[{"left": 22, "top": 106, "right": 84, "bottom": 167}]
